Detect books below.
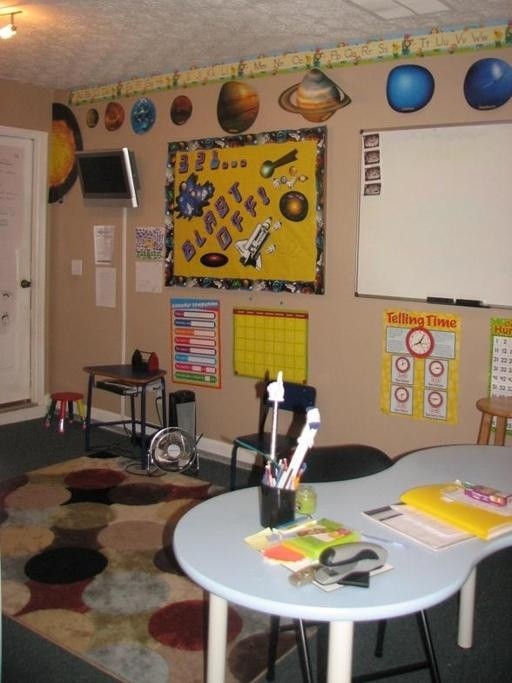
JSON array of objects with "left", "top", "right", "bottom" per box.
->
[
  {"left": 245, "top": 515, "right": 395, "bottom": 592},
  {"left": 360, "top": 477, "right": 512, "bottom": 551}
]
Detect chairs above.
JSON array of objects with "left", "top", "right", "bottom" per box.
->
[
  {"left": 230, "top": 379, "right": 316, "bottom": 491},
  {"left": 266, "top": 443, "right": 442, "bottom": 682}
]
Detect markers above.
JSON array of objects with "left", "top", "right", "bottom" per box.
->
[{"left": 426, "top": 297, "right": 482, "bottom": 308}]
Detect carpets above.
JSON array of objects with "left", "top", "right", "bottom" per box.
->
[{"left": 0, "top": 449, "right": 324, "bottom": 683}]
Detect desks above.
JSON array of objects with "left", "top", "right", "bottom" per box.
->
[
  {"left": 172, "top": 444, "right": 512, "bottom": 682},
  {"left": 83, "top": 365, "right": 167, "bottom": 470}
]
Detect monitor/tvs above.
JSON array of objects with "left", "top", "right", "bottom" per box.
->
[{"left": 72, "top": 144, "right": 142, "bottom": 209}]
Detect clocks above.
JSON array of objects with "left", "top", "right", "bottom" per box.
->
[
  {"left": 394, "top": 357, "right": 410, "bottom": 404},
  {"left": 427, "top": 361, "right": 444, "bottom": 407},
  {"left": 405, "top": 327, "right": 434, "bottom": 358}
]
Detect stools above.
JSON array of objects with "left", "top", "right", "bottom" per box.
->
[
  {"left": 44, "top": 392, "right": 87, "bottom": 433},
  {"left": 475, "top": 396, "right": 512, "bottom": 447}
]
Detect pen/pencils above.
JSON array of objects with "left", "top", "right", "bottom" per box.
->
[{"left": 262, "top": 458, "right": 308, "bottom": 490}]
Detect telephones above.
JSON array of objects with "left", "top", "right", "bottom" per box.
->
[{"left": 354, "top": 121, "right": 512, "bottom": 310}]
